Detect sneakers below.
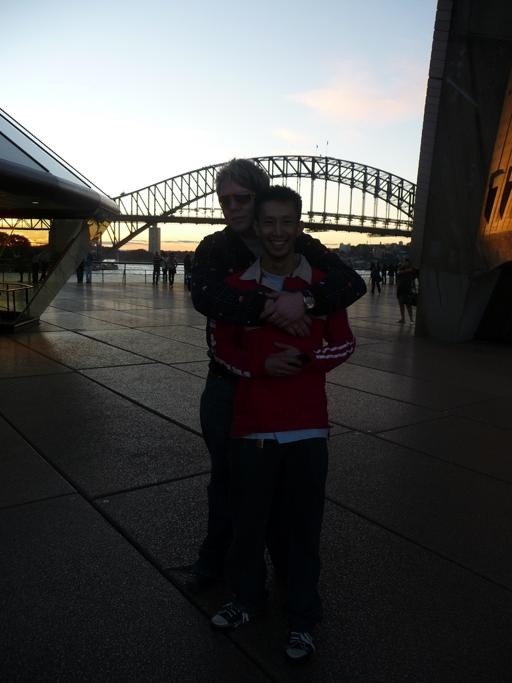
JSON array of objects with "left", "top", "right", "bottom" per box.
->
[
  {"left": 211, "top": 603, "right": 258, "bottom": 626},
  {"left": 286, "top": 632, "right": 315, "bottom": 658}
]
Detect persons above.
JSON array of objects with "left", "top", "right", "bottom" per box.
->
[
  {"left": 206, "top": 187, "right": 357, "bottom": 659},
  {"left": 369, "top": 257, "right": 417, "bottom": 326},
  {"left": 86, "top": 253, "right": 95, "bottom": 282},
  {"left": 198, "top": 160, "right": 366, "bottom": 599},
  {"left": 153, "top": 251, "right": 193, "bottom": 285},
  {"left": 77, "top": 260, "right": 83, "bottom": 283}
]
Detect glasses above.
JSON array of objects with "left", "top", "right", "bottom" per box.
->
[{"left": 219, "top": 192, "right": 255, "bottom": 207}]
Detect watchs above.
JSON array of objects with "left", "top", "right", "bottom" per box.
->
[{"left": 302, "top": 290, "right": 315, "bottom": 311}]
